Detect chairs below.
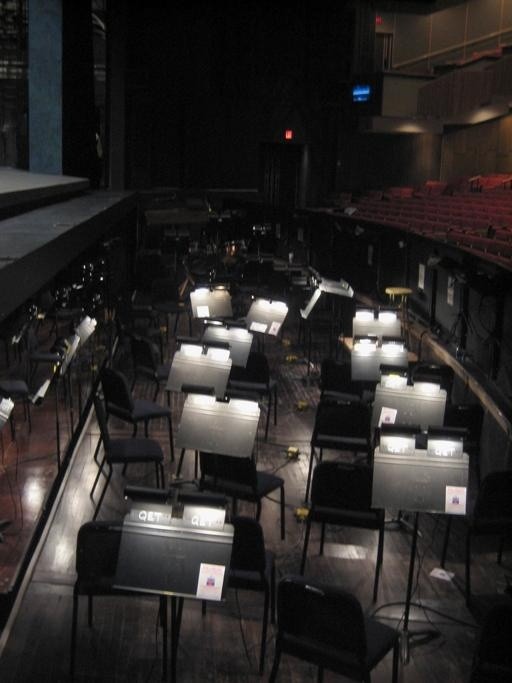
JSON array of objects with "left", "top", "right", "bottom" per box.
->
[{"left": 303, "top": 172, "right": 512, "bottom": 271}]
